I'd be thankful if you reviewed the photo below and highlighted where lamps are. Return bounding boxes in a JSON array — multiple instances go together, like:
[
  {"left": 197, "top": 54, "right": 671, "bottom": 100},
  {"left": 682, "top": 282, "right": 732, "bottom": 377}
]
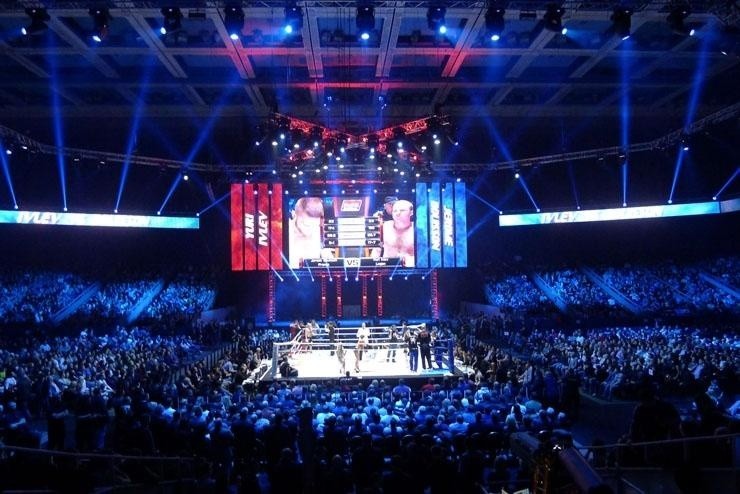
[{"left": 16, "top": 1, "right": 701, "bottom": 48}]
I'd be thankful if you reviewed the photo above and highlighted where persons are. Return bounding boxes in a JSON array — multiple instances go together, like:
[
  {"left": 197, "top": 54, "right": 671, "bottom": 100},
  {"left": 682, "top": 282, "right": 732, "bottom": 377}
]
[
  {"left": 371, "top": 195, "right": 400, "bottom": 223},
  {"left": 368, "top": 197, "right": 415, "bottom": 267},
  {"left": 357, "top": 334, "right": 365, "bottom": 361},
  {"left": 0, "top": 377, "right": 740, "bottom": 491},
  {"left": 334, "top": 341, "right": 348, "bottom": 372},
  {"left": 462, "top": 247, "right": 739, "bottom": 378},
  {"left": 356, "top": 322, "right": 371, "bottom": 354},
  {"left": 0, "top": 267, "right": 224, "bottom": 379},
  {"left": 324, "top": 320, "right": 336, "bottom": 357},
  {"left": 386, "top": 324, "right": 400, "bottom": 362},
  {"left": 287, "top": 194, "right": 338, "bottom": 268},
  {"left": 352, "top": 344, "right": 361, "bottom": 374},
  {"left": 223, "top": 316, "right": 322, "bottom": 381},
  {"left": 396, "top": 320, "right": 463, "bottom": 379}
]
